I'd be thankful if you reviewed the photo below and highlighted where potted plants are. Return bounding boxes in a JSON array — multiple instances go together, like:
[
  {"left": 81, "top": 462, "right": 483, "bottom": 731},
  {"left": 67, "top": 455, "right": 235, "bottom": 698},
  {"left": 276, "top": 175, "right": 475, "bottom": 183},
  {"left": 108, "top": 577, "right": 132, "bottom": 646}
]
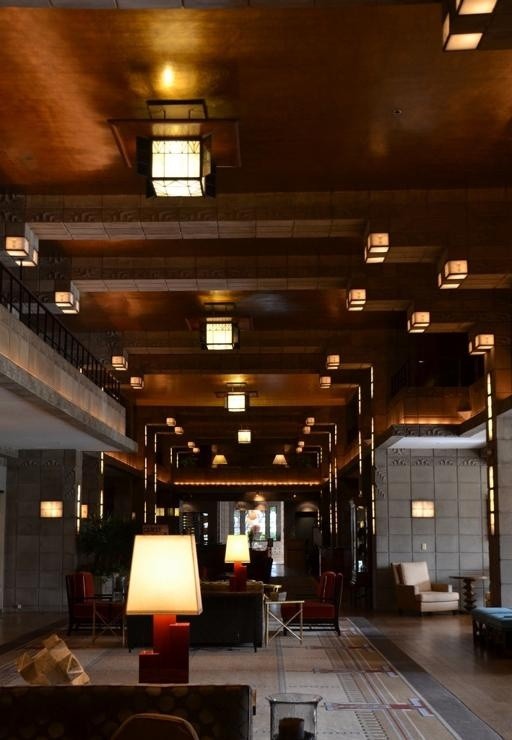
[{"left": 76, "top": 510, "right": 133, "bottom": 600}]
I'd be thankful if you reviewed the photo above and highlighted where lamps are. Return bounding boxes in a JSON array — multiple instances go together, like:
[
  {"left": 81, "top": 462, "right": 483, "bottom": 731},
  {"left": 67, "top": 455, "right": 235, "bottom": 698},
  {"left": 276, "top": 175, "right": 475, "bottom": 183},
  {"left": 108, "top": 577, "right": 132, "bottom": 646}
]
[
  {"left": 123, "top": 533, "right": 205, "bottom": 684},
  {"left": 4, "top": 221, "right": 44, "bottom": 273},
  {"left": 51, "top": 278, "right": 84, "bottom": 318},
  {"left": 223, "top": 532, "right": 252, "bottom": 580},
  {"left": 111, "top": 346, "right": 131, "bottom": 373},
  {"left": 441, "top": 0, "right": 500, "bottom": 53},
  {"left": 220, "top": 381, "right": 254, "bottom": 414},
  {"left": 132, "top": 101, "right": 221, "bottom": 201},
  {"left": 466, "top": 324, "right": 496, "bottom": 360},
  {"left": 296, "top": 354, "right": 345, "bottom": 457},
  {"left": 212, "top": 451, "right": 288, "bottom": 466},
  {"left": 197, "top": 300, "right": 241, "bottom": 353},
  {"left": 434, "top": 241, "right": 471, "bottom": 294},
  {"left": 405, "top": 301, "right": 432, "bottom": 339},
  {"left": 236, "top": 422, "right": 253, "bottom": 446},
  {"left": 343, "top": 279, "right": 366, "bottom": 313},
  {"left": 357, "top": 216, "right": 393, "bottom": 268},
  {"left": 130, "top": 369, "right": 201, "bottom": 456}
]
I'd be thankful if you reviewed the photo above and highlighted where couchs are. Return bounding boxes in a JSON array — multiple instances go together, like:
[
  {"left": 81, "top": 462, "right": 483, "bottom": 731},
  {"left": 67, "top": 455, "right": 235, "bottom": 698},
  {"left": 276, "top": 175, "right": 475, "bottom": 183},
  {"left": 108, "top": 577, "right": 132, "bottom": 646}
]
[{"left": 0, "top": 682, "right": 258, "bottom": 739}]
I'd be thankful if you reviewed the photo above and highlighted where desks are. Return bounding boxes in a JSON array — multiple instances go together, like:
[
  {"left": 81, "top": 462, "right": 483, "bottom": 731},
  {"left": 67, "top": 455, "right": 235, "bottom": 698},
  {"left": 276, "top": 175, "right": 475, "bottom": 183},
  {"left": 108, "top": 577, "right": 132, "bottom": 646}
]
[{"left": 448, "top": 571, "right": 489, "bottom": 613}]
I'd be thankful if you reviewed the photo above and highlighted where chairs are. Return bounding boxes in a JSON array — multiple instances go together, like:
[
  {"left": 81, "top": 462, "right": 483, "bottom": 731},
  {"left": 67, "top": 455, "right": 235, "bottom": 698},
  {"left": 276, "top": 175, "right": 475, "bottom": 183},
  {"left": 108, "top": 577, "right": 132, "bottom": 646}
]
[
  {"left": 63, "top": 567, "right": 345, "bottom": 654},
  {"left": 388, "top": 560, "right": 460, "bottom": 617},
  {"left": 470, "top": 603, "right": 511, "bottom": 657},
  {"left": 112, "top": 712, "right": 199, "bottom": 739}
]
